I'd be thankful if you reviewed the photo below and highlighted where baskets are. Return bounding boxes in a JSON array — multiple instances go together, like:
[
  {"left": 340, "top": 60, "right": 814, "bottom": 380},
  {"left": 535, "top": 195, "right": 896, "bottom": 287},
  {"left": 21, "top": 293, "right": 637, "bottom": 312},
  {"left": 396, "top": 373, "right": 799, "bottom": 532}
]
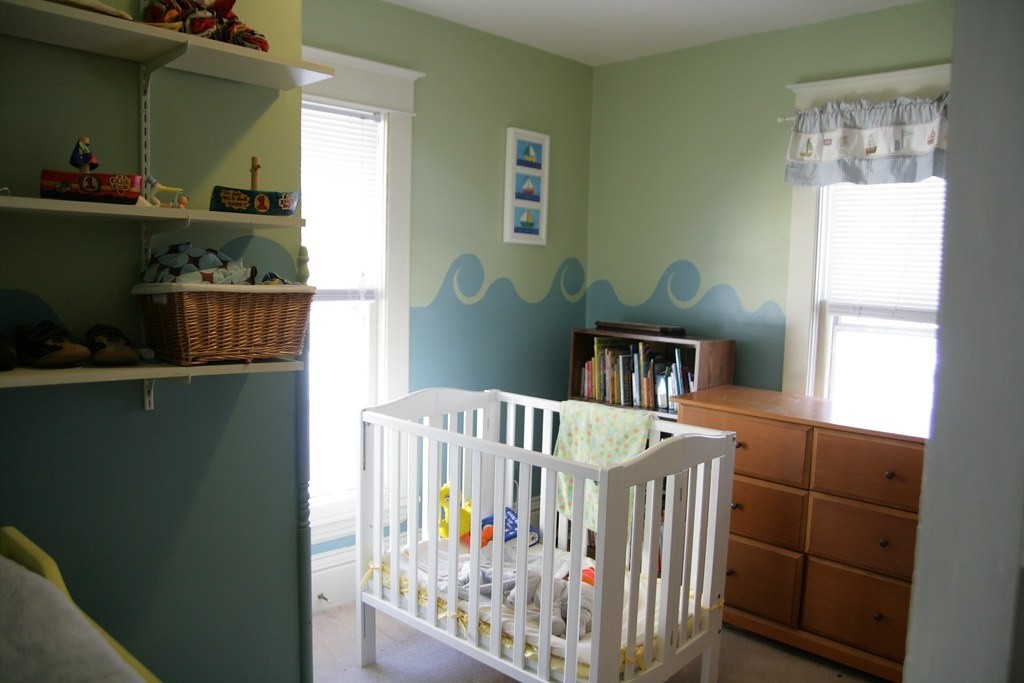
[{"left": 130, "top": 282, "right": 316, "bottom": 368}]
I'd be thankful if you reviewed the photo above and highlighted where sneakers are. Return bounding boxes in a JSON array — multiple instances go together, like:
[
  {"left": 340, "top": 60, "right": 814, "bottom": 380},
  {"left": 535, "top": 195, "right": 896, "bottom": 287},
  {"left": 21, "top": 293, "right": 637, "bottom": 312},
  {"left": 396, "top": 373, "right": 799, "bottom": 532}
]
[
  {"left": 16, "top": 321, "right": 92, "bottom": 368},
  {"left": 86, "top": 325, "right": 141, "bottom": 365}
]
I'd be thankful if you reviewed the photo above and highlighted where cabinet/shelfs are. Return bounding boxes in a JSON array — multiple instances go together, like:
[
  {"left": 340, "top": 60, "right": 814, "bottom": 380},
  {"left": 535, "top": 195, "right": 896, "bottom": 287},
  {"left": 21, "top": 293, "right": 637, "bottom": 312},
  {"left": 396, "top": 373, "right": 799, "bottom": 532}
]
[
  {"left": 1, "top": 1, "right": 335, "bottom": 411},
  {"left": 566, "top": 319, "right": 736, "bottom": 420},
  {"left": 671, "top": 381, "right": 930, "bottom": 683}
]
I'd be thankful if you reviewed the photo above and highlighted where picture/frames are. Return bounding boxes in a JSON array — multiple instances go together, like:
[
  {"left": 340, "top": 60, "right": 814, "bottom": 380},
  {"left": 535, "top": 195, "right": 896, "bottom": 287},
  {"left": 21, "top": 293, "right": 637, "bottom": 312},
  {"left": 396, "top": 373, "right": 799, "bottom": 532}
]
[{"left": 501, "top": 128, "right": 549, "bottom": 248}]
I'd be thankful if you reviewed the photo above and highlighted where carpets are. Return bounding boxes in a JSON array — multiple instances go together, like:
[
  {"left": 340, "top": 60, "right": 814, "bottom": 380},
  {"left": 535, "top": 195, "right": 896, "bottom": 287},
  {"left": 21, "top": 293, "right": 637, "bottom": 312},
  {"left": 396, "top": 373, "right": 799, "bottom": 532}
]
[{"left": 312, "top": 546, "right": 887, "bottom": 683}]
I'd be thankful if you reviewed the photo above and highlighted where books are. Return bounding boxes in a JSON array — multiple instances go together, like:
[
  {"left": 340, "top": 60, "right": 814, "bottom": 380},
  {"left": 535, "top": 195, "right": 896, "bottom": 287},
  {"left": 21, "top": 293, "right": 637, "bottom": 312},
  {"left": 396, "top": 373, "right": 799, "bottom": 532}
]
[{"left": 576, "top": 337, "right": 697, "bottom": 411}]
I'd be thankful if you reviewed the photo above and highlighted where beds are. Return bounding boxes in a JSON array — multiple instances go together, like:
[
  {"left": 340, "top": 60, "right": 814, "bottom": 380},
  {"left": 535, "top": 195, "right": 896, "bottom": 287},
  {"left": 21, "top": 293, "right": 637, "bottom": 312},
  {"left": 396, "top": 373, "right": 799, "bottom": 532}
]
[{"left": 357, "top": 386, "right": 738, "bottom": 683}]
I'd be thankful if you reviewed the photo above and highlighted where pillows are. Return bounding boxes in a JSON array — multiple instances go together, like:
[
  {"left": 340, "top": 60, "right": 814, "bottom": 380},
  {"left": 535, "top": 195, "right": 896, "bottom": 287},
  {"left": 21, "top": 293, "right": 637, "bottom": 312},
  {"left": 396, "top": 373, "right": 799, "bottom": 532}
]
[{"left": 481, "top": 506, "right": 540, "bottom": 553}]
